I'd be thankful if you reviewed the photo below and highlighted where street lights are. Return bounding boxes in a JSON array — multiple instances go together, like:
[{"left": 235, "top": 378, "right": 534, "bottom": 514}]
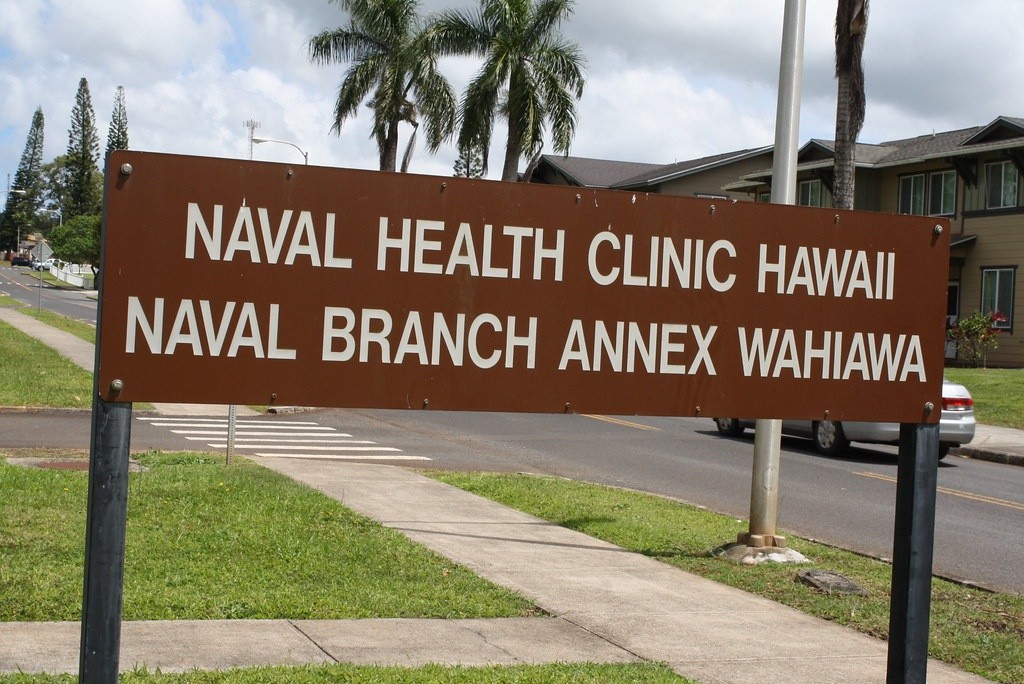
[{"left": 251, "top": 137, "right": 308, "bottom": 165}]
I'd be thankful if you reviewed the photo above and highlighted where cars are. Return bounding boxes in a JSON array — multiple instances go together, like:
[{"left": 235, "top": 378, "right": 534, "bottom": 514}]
[
  {"left": 33, "top": 257, "right": 57, "bottom": 272},
  {"left": 713, "top": 373, "right": 977, "bottom": 464},
  {"left": 11, "top": 257, "right": 30, "bottom": 267}
]
